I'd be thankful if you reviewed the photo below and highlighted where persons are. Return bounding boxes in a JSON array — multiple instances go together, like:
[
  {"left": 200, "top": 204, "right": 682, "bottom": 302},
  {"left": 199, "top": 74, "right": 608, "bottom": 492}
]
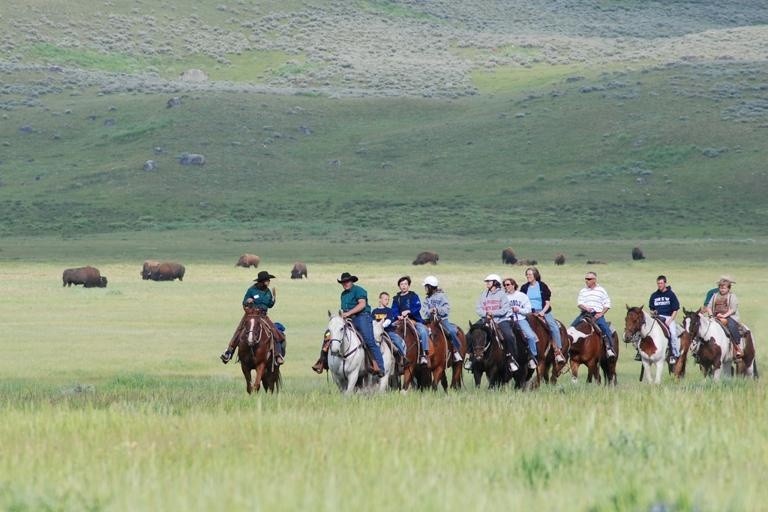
[
  {"left": 220, "top": 271, "right": 284, "bottom": 364},
  {"left": 702, "top": 276, "right": 736, "bottom": 313},
  {"left": 649, "top": 275, "right": 680, "bottom": 366},
  {"left": 312, "top": 266, "right": 567, "bottom": 377},
  {"left": 569, "top": 272, "right": 617, "bottom": 361},
  {"left": 706, "top": 280, "right": 744, "bottom": 358}
]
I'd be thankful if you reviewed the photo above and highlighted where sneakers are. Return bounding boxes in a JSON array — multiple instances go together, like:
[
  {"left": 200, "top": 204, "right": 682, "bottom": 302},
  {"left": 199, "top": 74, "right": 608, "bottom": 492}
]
[
  {"left": 275, "top": 353, "right": 285, "bottom": 367},
  {"left": 526, "top": 357, "right": 538, "bottom": 370},
  {"left": 605, "top": 349, "right": 616, "bottom": 361},
  {"left": 418, "top": 352, "right": 430, "bottom": 366},
  {"left": 452, "top": 350, "right": 465, "bottom": 364},
  {"left": 219, "top": 347, "right": 234, "bottom": 364},
  {"left": 552, "top": 352, "right": 566, "bottom": 365},
  {"left": 506, "top": 360, "right": 521, "bottom": 375}
]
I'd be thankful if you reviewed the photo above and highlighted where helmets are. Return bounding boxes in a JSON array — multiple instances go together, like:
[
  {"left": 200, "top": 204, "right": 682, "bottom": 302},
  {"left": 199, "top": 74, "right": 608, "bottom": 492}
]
[
  {"left": 421, "top": 275, "right": 440, "bottom": 287},
  {"left": 483, "top": 273, "right": 503, "bottom": 285}
]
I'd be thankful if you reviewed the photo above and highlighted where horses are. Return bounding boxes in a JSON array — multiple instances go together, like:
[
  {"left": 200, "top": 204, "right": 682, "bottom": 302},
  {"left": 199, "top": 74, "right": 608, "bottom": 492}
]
[
  {"left": 680, "top": 307, "right": 759, "bottom": 383},
  {"left": 567, "top": 316, "right": 620, "bottom": 386},
  {"left": 237, "top": 302, "right": 284, "bottom": 393},
  {"left": 322, "top": 307, "right": 468, "bottom": 396},
  {"left": 464, "top": 314, "right": 573, "bottom": 386},
  {"left": 621, "top": 302, "right": 684, "bottom": 385}
]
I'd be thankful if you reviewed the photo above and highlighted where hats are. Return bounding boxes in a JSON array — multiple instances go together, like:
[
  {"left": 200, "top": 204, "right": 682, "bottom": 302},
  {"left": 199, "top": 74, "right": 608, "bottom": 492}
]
[
  {"left": 336, "top": 272, "right": 358, "bottom": 283},
  {"left": 252, "top": 270, "right": 276, "bottom": 282}
]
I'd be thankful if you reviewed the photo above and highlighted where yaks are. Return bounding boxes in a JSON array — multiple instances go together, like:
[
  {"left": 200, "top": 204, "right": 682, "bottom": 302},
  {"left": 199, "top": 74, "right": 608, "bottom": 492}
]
[
  {"left": 412, "top": 251, "right": 440, "bottom": 266},
  {"left": 61, "top": 266, "right": 108, "bottom": 288},
  {"left": 141, "top": 259, "right": 186, "bottom": 281},
  {"left": 234, "top": 253, "right": 261, "bottom": 269},
  {"left": 502, "top": 247, "right": 538, "bottom": 265},
  {"left": 554, "top": 254, "right": 566, "bottom": 265},
  {"left": 632, "top": 248, "right": 645, "bottom": 261},
  {"left": 290, "top": 263, "right": 308, "bottom": 278}
]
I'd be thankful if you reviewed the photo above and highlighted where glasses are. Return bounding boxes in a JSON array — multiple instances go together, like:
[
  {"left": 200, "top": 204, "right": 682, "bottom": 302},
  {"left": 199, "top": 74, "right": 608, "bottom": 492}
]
[
  {"left": 504, "top": 284, "right": 511, "bottom": 287},
  {"left": 584, "top": 277, "right": 594, "bottom": 281}
]
